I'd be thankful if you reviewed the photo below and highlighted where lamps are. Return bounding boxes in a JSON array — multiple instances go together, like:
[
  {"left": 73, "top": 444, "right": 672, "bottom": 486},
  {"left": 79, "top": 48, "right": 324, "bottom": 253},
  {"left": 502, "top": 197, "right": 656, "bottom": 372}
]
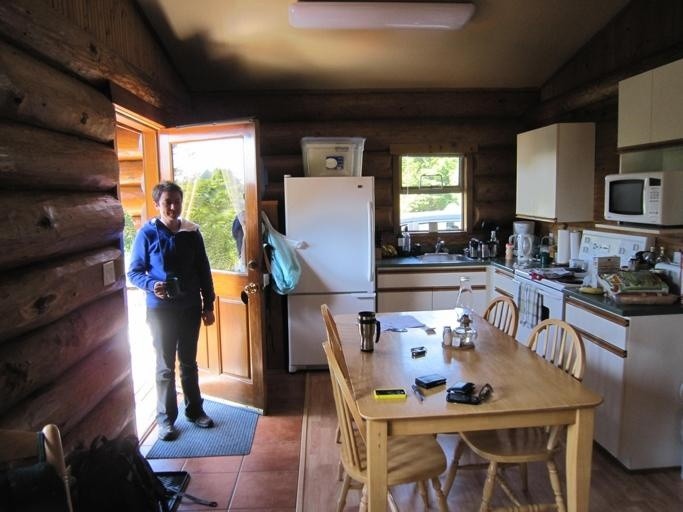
[{"left": 285, "top": 0, "right": 477, "bottom": 34}]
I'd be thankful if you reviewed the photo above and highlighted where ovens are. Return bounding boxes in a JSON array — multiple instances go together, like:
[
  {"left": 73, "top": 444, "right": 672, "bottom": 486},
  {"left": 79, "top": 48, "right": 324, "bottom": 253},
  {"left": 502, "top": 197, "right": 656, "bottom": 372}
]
[{"left": 512, "top": 275, "right": 564, "bottom": 368}]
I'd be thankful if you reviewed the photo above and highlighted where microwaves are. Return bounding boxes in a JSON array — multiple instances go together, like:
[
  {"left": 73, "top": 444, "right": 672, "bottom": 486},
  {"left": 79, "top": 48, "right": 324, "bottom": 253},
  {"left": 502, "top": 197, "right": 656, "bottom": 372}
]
[{"left": 602, "top": 170, "right": 682, "bottom": 227}]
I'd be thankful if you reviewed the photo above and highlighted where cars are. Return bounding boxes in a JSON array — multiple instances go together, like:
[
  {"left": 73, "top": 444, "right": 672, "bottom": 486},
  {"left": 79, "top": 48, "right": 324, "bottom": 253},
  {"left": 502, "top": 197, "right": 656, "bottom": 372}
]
[{"left": 399, "top": 214, "right": 460, "bottom": 232}]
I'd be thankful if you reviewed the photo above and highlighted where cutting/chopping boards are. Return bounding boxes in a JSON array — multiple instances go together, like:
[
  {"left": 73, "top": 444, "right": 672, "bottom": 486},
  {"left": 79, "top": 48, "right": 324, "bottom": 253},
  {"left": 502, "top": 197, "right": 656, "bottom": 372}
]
[{"left": 611, "top": 293, "right": 678, "bottom": 305}]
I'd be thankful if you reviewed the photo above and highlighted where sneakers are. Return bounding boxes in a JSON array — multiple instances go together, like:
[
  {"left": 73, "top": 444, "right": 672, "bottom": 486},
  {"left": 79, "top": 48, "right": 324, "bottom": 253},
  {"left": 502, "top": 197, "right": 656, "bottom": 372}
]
[
  {"left": 185, "top": 406, "right": 213, "bottom": 427},
  {"left": 159, "top": 422, "right": 176, "bottom": 439}
]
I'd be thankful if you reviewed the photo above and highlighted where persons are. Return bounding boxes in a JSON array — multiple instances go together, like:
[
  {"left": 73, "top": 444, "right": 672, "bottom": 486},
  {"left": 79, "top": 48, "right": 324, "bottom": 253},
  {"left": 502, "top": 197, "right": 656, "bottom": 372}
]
[
  {"left": 231, "top": 193, "right": 249, "bottom": 272},
  {"left": 126, "top": 180, "right": 216, "bottom": 443}
]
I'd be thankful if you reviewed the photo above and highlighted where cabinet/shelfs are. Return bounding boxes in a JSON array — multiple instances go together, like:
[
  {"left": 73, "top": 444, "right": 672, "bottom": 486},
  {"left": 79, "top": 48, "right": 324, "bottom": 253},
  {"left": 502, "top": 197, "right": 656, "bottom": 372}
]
[
  {"left": 561, "top": 297, "right": 681, "bottom": 478},
  {"left": 616, "top": 58, "right": 682, "bottom": 153},
  {"left": 514, "top": 122, "right": 596, "bottom": 225},
  {"left": 375, "top": 262, "right": 489, "bottom": 314},
  {"left": 490, "top": 263, "right": 521, "bottom": 338}
]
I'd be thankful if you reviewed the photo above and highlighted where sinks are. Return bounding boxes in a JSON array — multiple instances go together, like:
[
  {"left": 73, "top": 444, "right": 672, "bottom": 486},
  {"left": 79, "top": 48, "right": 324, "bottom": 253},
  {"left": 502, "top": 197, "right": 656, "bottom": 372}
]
[{"left": 415, "top": 253, "right": 477, "bottom": 264}]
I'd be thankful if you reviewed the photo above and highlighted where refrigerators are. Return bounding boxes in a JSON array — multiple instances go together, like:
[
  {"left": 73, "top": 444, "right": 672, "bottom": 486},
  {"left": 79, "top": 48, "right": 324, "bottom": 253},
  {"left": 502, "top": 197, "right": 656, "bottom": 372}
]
[{"left": 282, "top": 174, "right": 376, "bottom": 373}]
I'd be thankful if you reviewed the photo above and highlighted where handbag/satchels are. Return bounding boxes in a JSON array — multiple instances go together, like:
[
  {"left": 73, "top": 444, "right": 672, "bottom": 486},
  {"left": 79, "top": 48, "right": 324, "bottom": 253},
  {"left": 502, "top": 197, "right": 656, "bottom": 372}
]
[{"left": 7, "top": 462, "right": 69, "bottom": 512}]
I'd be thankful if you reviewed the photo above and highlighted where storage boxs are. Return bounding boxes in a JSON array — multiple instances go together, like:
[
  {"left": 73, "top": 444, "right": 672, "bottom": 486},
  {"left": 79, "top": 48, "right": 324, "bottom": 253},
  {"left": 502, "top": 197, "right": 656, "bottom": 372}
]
[{"left": 298, "top": 134, "right": 366, "bottom": 177}]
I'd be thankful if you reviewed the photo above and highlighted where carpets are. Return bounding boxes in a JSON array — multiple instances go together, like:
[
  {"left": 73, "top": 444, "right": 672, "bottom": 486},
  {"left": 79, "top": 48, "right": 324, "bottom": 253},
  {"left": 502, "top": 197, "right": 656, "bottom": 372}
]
[{"left": 144, "top": 386, "right": 265, "bottom": 460}]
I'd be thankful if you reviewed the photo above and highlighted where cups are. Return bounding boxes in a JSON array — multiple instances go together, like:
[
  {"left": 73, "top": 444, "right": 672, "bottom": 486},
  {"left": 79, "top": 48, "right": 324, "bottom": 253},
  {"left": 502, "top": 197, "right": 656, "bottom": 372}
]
[
  {"left": 162, "top": 277, "right": 180, "bottom": 300},
  {"left": 357, "top": 310, "right": 381, "bottom": 352},
  {"left": 540, "top": 252, "right": 550, "bottom": 264},
  {"left": 568, "top": 259, "right": 576, "bottom": 268}
]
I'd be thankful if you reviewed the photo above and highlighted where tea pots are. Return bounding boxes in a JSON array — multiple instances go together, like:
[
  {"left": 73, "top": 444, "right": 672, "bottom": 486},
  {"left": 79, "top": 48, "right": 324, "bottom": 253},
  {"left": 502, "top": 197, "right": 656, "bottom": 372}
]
[{"left": 538, "top": 235, "right": 557, "bottom": 261}]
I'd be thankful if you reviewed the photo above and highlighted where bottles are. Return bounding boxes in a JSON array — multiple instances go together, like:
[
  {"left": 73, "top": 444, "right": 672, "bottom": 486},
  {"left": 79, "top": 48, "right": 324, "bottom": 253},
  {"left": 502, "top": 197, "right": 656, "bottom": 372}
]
[
  {"left": 396, "top": 225, "right": 411, "bottom": 255},
  {"left": 468, "top": 241, "right": 488, "bottom": 259},
  {"left": 435, "top": 241, "right": 440, "bottom": 254},
  {"left": 646, "top": 246, "right": 669, "bottom": 266},
  {"left": 489, "top": 231, "right": 497, "bottom": 258},
  {"left": 504, "top": 243, "right": 513, "bottom": 260},
  {"left": 455, "top": 278, "right": 477, "bottom": 328},
  {"left": 442, "top": 326, "right": 453, "bottom": 346}
]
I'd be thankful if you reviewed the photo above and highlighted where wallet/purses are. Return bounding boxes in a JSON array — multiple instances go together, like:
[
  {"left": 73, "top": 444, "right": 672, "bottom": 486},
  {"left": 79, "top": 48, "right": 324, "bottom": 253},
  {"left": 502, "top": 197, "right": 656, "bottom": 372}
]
[{"left": 447, "top": 383, "right": 476, "bottom": 393}]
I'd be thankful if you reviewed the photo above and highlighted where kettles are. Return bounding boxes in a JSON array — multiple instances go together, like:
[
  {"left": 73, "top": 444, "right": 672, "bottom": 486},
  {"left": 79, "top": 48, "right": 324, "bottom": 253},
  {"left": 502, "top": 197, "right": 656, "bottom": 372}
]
[{"left": 517, "top": 233, "right": 536, "bottom": 261}]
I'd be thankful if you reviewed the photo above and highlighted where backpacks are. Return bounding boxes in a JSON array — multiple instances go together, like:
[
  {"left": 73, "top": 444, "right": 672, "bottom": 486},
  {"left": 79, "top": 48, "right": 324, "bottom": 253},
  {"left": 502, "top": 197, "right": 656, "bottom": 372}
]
[{"left": 90, "top": 436, "right": 167, "bottom": 500}]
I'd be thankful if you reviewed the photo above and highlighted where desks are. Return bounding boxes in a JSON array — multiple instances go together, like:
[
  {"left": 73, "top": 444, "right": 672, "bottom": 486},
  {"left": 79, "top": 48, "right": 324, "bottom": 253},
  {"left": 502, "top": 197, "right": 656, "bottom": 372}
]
[{"left": 333, "top": 306, "right": 603, "bottom": 512}]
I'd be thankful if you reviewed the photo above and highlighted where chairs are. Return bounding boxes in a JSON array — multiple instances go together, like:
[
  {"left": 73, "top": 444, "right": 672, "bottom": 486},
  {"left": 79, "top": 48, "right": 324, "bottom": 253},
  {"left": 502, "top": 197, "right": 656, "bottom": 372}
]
[
  {"left": 319, "top": 338, "right": 451, "bottom": 510},
  {"left": 1, "top": 424, "right": 78, "bottom": 512},
  {"left": 318, "top": 302, "right": 438, "bottom": 449},
  {"left": 439, "top": 316, "right": 588, "bottom": 510},
  {"left": 480, "top": 295, "right": 521, "bottom": 342}
]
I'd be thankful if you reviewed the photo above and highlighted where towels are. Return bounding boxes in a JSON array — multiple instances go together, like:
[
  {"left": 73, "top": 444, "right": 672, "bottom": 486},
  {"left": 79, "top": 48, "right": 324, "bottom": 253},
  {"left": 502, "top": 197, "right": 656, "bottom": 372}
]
[{"left": 514, "top": 282, "right": 542, "bottom": 330}]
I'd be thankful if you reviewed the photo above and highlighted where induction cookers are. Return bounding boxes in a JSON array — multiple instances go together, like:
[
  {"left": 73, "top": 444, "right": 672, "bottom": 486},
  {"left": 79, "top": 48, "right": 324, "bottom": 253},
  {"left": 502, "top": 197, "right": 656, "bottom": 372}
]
[{"left": 515, "top": 267, "right": 589, "bottom": 286}]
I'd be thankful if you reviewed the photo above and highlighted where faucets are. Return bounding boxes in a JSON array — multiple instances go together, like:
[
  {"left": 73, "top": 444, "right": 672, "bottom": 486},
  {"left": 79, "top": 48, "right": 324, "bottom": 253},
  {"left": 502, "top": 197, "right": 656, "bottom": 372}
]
[{"left": 434, "top": 239, "right": 446, "bottom": 254}]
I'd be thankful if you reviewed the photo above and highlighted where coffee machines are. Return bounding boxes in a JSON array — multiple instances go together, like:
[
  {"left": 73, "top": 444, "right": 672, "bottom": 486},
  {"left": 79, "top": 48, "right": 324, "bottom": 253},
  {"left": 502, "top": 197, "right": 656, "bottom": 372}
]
[{"left": 508, "top": 220, "right": 535, "bottom": 256}]
